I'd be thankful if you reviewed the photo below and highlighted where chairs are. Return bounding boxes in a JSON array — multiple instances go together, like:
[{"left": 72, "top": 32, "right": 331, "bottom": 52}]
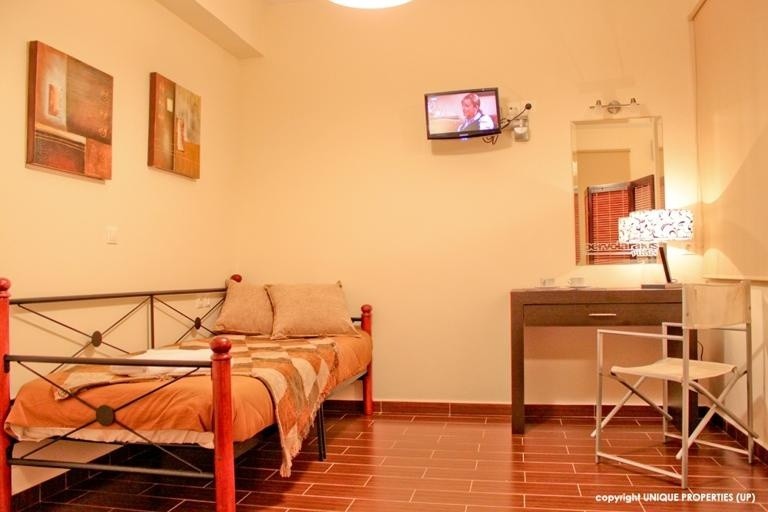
[{"left": 591, "top": 281, "right": 759, "bottom": 489}]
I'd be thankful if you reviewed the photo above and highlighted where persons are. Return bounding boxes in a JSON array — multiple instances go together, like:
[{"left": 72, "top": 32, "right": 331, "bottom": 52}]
[{"left": 457, "top": 92, "right": 494, "bottom": 133}]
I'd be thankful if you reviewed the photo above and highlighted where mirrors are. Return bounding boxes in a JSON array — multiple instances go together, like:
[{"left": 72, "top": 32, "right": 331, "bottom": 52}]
[{"left": 571, "top": 115, "right": 667, "bottom": 266}]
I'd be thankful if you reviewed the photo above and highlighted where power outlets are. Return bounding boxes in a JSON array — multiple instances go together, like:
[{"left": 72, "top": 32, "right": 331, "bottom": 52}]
[{"left": 507, "top": 103, "right": 532, "bottom": 143}]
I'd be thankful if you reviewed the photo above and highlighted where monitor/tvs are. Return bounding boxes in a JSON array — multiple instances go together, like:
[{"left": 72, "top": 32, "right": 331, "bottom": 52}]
[{"left": 424, "top": 88, "right": 501, "bottom": 140}]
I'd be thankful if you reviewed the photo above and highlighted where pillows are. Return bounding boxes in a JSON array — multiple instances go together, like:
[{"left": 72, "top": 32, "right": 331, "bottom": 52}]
[
  {"left": 264, "top": 280, "right": 361, "bottom": 340},
  {"left": 211, "top": 279, "right": 272, "bottom": 335}
]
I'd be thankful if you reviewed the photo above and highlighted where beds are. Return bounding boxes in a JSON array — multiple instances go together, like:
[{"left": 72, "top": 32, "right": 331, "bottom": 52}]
[{"left": 0, "top": 274, "right": 372, "bottom": 512}]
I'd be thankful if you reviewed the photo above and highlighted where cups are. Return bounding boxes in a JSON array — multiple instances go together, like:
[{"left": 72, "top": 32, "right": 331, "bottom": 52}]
[
  {"left": 566, "top": 277, "right": 585, "bottom": 287},
  {"left": 540, "top": 277, "right": 556, "bottom": 287}
]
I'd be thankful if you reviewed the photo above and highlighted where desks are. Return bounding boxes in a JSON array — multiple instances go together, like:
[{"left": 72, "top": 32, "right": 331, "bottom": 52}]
[{"left": 511, "top": 289, "right": 697, "bottom": 436}]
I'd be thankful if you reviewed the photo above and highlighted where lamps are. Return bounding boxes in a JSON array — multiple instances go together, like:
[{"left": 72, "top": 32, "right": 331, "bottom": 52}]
[
  {"left": 590, "top": 98, "right": 642, "bottom": 119},
  {"left": 618, "top": 217, "right": 629, "bottom": 245},
  {"left": 630, "top": 209, "right": 693, "bottom": 286}
]
